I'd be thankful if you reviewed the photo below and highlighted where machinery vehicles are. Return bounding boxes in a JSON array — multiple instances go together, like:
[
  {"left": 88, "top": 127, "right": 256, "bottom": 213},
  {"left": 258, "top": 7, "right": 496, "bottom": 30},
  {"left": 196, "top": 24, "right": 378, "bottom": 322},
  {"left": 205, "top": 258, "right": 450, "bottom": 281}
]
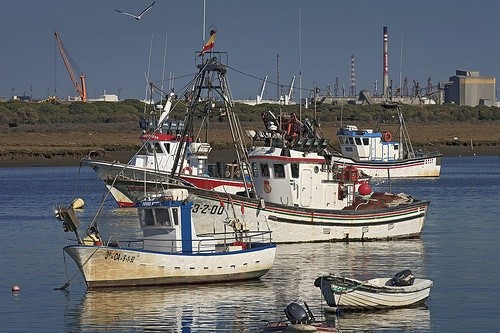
[{"left": 53, "top": 30, "right": 88, "bottom": 104}]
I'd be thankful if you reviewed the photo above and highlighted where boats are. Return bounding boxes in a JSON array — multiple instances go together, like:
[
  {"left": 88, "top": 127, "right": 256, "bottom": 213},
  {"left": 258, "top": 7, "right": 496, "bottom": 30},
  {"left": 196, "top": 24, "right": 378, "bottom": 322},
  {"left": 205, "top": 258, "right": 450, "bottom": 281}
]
[{"left": 313, "top": 268, "right": 435, "bottom": 312}]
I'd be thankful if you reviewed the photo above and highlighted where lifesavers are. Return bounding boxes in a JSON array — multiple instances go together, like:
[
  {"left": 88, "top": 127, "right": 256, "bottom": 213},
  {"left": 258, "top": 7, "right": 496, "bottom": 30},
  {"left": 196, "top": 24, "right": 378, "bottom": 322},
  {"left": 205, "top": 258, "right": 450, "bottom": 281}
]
[
  {"left": 181, "top": 166, "right": 192, "bottom": 176},
  {"left": 382, "top": 131, "right": 392, "bottom": 142}
]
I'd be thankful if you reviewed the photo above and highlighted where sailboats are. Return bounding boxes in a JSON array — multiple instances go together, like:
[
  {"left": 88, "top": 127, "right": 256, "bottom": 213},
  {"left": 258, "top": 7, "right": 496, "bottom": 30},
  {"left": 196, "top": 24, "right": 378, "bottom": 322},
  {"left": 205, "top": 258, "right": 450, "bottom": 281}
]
[
  {"left": 162, "top": 25, "right": 431, "bottom": 240},
  {"left": 52, "top": 150, "right": 278, "bottom": 291},
  {"left": 88, "top": 73, "right": 257, "bottom": 208},
  {"left": 329, "top": 91, "right": 444, "bottom": 179}
]
